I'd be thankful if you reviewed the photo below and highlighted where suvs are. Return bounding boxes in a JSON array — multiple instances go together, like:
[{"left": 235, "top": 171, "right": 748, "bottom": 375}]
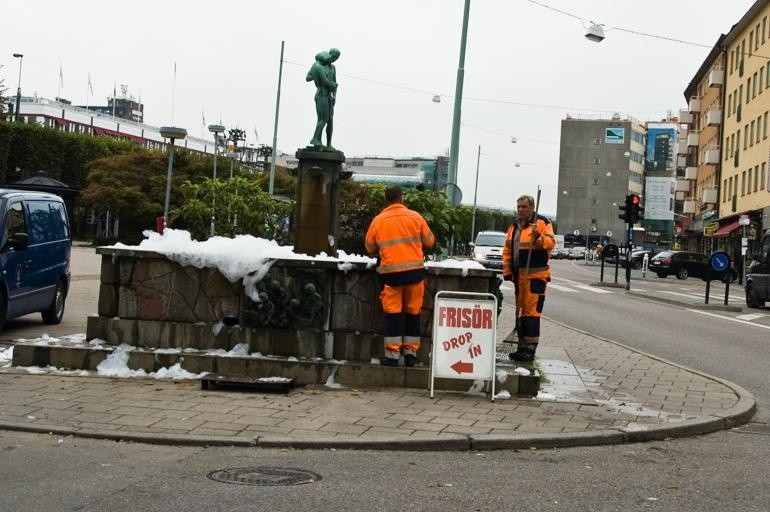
[{"left": 468, "top": 231, "right": 507, "bottom": 273}]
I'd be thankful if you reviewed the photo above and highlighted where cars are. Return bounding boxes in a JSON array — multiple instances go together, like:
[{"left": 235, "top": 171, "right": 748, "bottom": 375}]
[
  {"left": 550, "top": 247, "right": 661, "bottom": 270},
  {"left": 744, "top": 227, "right": 770, "bottom": 308},
  {"left": 649, "top": 250, "right": 737, "bottom": 284}
]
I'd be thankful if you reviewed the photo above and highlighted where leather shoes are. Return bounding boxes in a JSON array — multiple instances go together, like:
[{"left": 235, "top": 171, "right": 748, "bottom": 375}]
[
  {"left": 404, "top": 355, "right": 417, "bottom": 366},
  {"left": 509, "top": 348, "right": 534, "bottom": 361},
  {"left": 380, "top": 358, "right": 398, "bottom": 366}
]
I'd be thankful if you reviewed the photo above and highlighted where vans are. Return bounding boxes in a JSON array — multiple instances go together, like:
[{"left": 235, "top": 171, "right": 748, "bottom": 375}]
[{"left": 0, "top": 189, "right": 72, "bottom": 333}]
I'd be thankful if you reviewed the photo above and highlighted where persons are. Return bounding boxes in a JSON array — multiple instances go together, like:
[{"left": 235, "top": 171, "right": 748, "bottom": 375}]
[
  {"left": 502, "top": 195, "right": 555, "bottom": 360},
  {"left": 306, "top": 48, "right": 340, "bottom": 148},
  {"left": 243, "top": 274, "right": 324, "bottom": 329},
  {"left": 364, "top": 185, "right": 435, "bottom": 366}
]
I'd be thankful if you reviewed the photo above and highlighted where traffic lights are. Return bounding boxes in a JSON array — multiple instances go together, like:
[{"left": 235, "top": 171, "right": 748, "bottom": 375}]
[
  {"left": 634, "top": 200, "right": 645, "bottom": 223},
  {"left": 618, "top": 196, "right": 631, "bottom": 223},
  {"left": 627, "top": 193, "right": 640, "bottom": 225}
]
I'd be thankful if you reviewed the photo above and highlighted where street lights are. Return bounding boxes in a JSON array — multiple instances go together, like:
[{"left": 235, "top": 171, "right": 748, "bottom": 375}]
[
  {"left": 13, "top": 53, "right": 23, "bottom": 122},
  {"left": 207, "top": 124, "right": 225, "bottom": 237},
  {"left": 227, "top": 153, "right": 239, "bottom": 225},
  {"left": 159, "top": 127, "right": 187, "bottom": 229}
]
